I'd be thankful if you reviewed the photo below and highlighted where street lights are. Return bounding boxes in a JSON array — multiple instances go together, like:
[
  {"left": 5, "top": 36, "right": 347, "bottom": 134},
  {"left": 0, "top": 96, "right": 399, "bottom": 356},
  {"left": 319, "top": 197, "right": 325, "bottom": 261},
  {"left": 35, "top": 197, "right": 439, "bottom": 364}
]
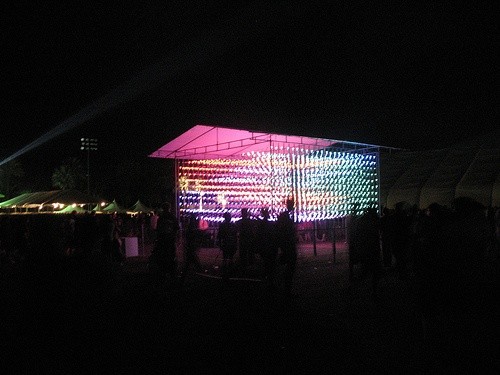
[{"left": 79, "top": 137, "right": 99, "bottom": 214}]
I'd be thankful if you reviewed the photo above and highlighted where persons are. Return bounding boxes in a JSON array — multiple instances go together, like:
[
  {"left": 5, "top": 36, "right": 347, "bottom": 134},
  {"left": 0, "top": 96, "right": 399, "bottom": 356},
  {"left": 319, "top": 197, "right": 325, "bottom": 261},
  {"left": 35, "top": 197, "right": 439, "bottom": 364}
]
[
  {"left": 155, "top": 202, "right": 180, "bottom": 269},
  {"left": 1, "top": 200, "right": 159, "bottom": 264},
  {"left": 345, "top": 199, "right": 500, "bottom": 270},
  {"left": 253, "top": 207, "right": 276, "bottom": 259},
  {"left": 217, "top": 211, "right": 238, "bottom": 270},
  {"left": 236, "top": 208, "right": 256, "bottom": 266},
  {"left": 276, "top": 196, "right": 299, "bottom": 293},
  {"left": 181, "top": 216, "right": 209, "bottom": 272}
]
